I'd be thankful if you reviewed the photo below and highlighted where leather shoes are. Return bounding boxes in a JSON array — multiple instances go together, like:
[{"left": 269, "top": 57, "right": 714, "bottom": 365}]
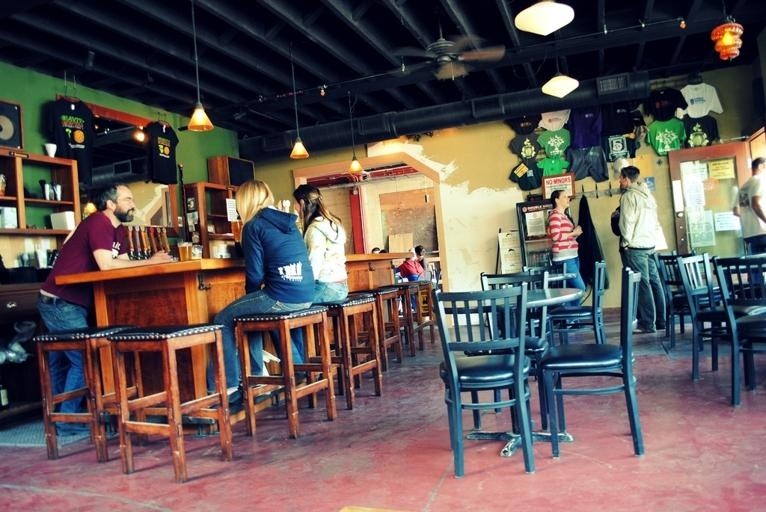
[{"left": 56, "top": 422, "right": 90, "bottom": 435}]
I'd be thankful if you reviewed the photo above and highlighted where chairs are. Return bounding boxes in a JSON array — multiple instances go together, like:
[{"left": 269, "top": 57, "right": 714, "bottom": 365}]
[
  {"left": 479, "top": 262, "right": 611, "bottom": 384},
  {"left": 545, "top": 267, "right": 644, "bottom": 460},
  {"left": 431, "top": 281, "right": 539, "bottom": 477},
  {"left": 655, "top": 248, "right": 766, "bottom": 406}
]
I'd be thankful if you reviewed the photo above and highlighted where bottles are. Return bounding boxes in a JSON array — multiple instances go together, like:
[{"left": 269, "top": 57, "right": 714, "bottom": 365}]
[{"left": 528, "top": 247, "right": 550, "bottom": 267}]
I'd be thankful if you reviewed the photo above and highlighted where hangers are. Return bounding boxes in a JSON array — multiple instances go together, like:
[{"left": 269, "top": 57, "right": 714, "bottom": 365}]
[
  {"left": 64, "top": 82, "right": 80, "bottom": 101},
  {"left": 156, "top": 110, "right": 169, "bottom": 125}
]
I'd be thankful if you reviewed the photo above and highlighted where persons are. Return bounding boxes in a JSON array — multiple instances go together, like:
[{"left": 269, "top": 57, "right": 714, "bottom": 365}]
[
  {"left": 371, "top": 245, "right": 428, "bottom": 310},
  {"left": 733, "top": 157, "right": 766, "bottom": 254},
  {"left": 610, "top": 185, "right": 628, "bottom": 270},
  {"left": 208, "top": 179, "right": 313, "bottom": 402},
  {"left": 611, "top": 166, "right": 665, "bottom": 332},
  {"left": 293, "top": 183, "right": 348, "bottom": 305},
  {"left": 35, "top": 181, "right": 172, "bottom": 435},
  {"left": 548, "top": 190, "right": 585, "bottom": 328}
]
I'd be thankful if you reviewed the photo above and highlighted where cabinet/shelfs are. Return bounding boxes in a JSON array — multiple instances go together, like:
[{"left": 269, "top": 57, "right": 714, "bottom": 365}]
[
  {"left": 181, "top": 155, "right": 256, "bottom": 258},
  {"left": 0, "top": 279, "right": 47, "bottom": 429},
  {"left": 0, "top": 146, "right": 81, "bottom": 237}
]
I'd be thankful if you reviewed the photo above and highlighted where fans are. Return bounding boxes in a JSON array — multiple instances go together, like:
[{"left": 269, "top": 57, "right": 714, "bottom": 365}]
[{"left": 387, "top": 27, "right": 504, "bottom": 79}]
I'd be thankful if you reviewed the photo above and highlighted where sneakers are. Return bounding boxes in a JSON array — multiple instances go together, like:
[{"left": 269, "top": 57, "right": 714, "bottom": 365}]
[{"left": 632, "top": 327, "right": 652, "bottom": 334}]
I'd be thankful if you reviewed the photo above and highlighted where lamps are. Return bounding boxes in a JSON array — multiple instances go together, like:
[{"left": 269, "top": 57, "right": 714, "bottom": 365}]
[
  {"left": 514, "top": 0, "right": 575, "bottom": 36},
  {"left": 289, "top": 55, "right": 311, "bottom": 160},
  {"left": 187, "top": 2, "right": 215, "bottom": 136},
  {"left": 541, "top": 69, "right": 581, "bottom": 100},
  {"left": 347, "top": 92, "right": 363, "bottom": 175},
  {"left": 708, "top": 10, "right": 744, "bottom": 63}
]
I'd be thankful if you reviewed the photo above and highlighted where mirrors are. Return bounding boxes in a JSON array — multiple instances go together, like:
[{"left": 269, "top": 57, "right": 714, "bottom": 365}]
[{"left": 51, "top": 93, "right": 178, "bottom": 237}]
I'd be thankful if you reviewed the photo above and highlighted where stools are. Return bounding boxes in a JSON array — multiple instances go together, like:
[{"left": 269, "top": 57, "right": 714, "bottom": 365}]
[
  {"left": 343, "top": 285, "right": 403, "bottom": 371},
  {"left": 310, "top": 288, "right": 383, "bottom": 412},
  {"left": 387, "top": 279, "right": 436, "bottom": 351},
  {"left": 111, "top": 324, "right": 232, "bottom": 485},
  {"left": 376, "top": 282, "right": 424, "bottom": 360},
  {"left": 230, "top": 304, "right": 339, "bottom": 441},
  {"left": 30, "top": 324, "right": 146, "bottom": 463}
]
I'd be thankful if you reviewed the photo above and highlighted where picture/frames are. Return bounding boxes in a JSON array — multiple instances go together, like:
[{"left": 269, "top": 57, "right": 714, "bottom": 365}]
[{"left": 0, "top": 98, "right": 28, "bottom": 152}]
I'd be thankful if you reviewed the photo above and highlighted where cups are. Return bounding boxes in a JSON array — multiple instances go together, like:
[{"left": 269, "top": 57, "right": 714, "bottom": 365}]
[
  {"left": 192, "top": 245, "right": 202, "bottom": 259},
  {"left": 20, "top": 253, "right": 29, "bottom": 267},
  {"left": 177, "top": 241, "right": 192, "bottom": 262},
  {"left": 44, "top": 143, "right": 57, "bottom": 159},
  {"left": 34, "top": 249, "right": 47, "bottom": 268}
]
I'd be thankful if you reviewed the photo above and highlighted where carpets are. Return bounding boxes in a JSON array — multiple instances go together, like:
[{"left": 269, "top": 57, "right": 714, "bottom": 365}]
[{"left": 0, "top": 408, "right": 102, "bottom": 449}]
